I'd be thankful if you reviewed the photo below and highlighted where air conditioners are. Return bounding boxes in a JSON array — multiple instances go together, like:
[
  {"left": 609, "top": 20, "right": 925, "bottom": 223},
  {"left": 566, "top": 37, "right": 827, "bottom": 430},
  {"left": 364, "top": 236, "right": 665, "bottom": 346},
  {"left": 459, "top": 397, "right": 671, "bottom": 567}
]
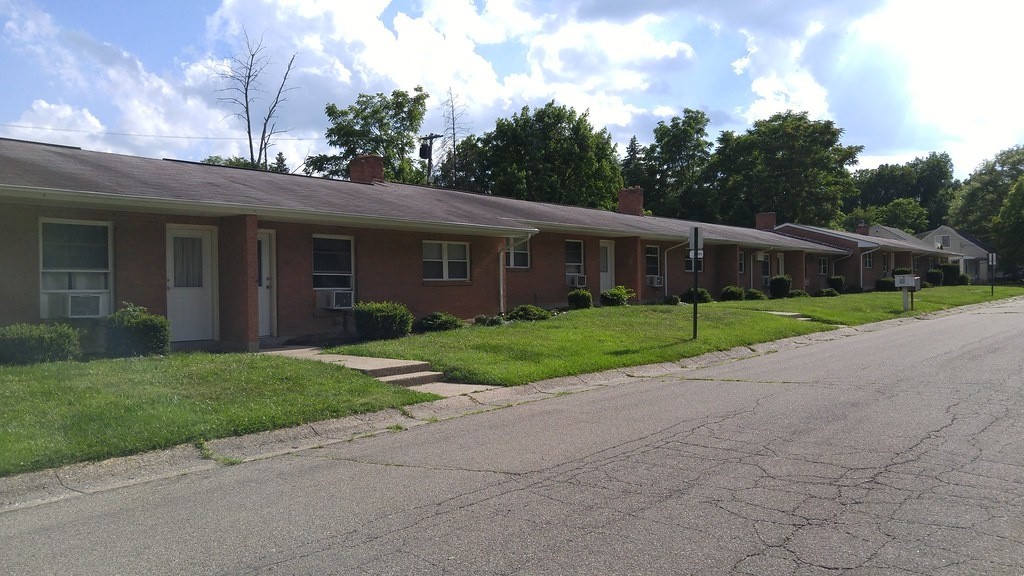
[
  {"left": 321, "top": 290, "right": 354, "bottom": 311},
  {"left": 56, "top": 292, "right": 100, "bottom": 318},
  {"left": 567, "top": 273, "right": 586, "bottom": 287},
  {"left": 646, "top": 274, "right": 664, "bottom": 288}
]
